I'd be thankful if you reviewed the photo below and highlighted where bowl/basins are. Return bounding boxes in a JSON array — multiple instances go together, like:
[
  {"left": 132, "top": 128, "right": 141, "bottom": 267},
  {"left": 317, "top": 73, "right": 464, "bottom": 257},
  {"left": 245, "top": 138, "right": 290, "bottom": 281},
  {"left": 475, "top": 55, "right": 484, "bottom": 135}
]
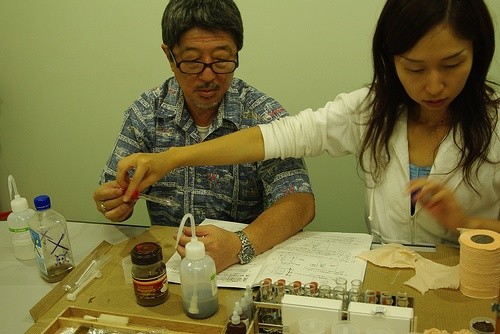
[{"left": 470, "top": 316, "right": 495, "bottom": 334}]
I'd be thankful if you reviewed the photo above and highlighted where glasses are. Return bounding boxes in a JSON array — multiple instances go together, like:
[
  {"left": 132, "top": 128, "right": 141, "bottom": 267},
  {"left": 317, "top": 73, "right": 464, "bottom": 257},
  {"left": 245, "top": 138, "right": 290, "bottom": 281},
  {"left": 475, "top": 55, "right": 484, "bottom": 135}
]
[{"left": 168, "top": 49, "right": 239, "bottom": 74}]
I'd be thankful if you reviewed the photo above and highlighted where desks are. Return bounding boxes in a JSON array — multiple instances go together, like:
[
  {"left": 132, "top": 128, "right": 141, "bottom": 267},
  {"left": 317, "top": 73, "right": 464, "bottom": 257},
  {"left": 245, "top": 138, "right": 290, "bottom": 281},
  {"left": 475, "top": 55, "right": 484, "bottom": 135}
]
[{"left": 0, "top": 222, "right": 500, "bottom": 334}]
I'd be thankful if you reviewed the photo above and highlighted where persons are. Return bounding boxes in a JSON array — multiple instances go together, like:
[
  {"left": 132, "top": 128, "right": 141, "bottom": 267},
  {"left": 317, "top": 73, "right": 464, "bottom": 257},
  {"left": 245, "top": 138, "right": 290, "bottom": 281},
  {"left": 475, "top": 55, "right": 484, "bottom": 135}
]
[
  {"left": 93, "top": 0, "right": 316, "bottom": 274},
  {"left": 116, "top": 0, "right": 500, "bottom": 248}
]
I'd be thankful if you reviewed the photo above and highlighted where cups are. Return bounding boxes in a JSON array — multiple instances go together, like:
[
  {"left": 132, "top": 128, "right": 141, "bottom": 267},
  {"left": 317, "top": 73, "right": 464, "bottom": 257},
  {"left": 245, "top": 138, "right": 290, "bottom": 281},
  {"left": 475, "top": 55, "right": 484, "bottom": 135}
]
[{"left": 298, "top": 318, "right": 396, "bottom": 334}]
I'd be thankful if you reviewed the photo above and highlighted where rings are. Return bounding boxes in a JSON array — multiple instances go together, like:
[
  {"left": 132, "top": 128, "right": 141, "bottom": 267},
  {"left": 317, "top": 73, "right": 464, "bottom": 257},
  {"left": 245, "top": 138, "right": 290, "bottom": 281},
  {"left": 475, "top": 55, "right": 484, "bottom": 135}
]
[{"left": 101, "top": 201, "right": 107, "bottom": 212}]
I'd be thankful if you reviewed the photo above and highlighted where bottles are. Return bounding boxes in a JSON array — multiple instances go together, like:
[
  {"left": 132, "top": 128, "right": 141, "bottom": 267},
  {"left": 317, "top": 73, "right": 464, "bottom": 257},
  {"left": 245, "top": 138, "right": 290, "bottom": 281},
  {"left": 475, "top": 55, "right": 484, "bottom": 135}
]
[
  {"left": 179, "top": 236, "right": 219, "bottom": 319},
  {"left": 27, "top": 195, "right": 76, "bottom": 284},
  {"left": 131, "top": 242, "right": 169, "bottom": 306},
  {"left": 7, "top": 194, "right": 37, "bottom": 261}
]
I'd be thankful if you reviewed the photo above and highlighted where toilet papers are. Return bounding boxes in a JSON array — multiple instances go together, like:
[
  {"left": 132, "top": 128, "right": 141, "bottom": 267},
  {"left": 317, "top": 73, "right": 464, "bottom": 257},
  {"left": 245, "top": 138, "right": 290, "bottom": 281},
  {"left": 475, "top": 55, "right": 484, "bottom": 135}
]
[{"left": 456, "top": 228, "right": 500, "bottom": 300}]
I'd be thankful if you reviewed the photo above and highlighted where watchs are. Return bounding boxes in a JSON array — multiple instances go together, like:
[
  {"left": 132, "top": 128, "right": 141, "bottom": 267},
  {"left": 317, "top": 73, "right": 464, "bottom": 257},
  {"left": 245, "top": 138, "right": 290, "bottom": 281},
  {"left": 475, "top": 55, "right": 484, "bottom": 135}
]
[{"left": 235, "top": 231, "right": 254, "bottom": 265}]
